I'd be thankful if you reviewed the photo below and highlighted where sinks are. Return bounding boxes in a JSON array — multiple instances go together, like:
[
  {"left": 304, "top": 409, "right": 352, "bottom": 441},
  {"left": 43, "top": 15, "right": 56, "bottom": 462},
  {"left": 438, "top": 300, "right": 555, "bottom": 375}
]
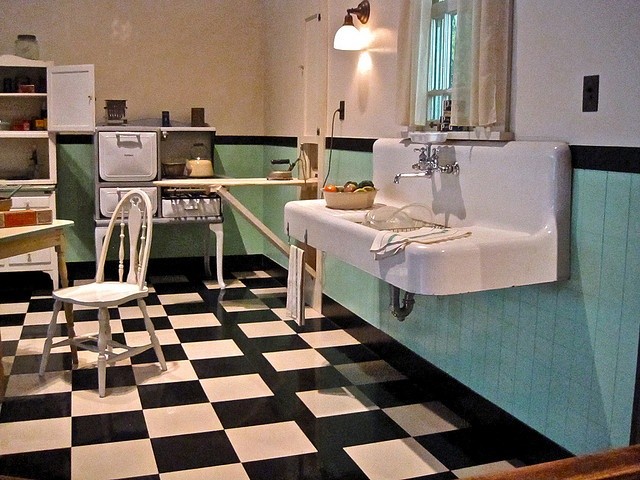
[{"left": 285, "top": 138, "right": 570, "bottom": 294}]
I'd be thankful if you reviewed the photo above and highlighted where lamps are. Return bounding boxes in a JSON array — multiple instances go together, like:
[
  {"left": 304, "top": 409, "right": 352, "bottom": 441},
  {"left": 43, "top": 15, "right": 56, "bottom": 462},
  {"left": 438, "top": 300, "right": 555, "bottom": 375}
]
[{"left": 334, "top": 0, "right": 371, "bottom": 52}]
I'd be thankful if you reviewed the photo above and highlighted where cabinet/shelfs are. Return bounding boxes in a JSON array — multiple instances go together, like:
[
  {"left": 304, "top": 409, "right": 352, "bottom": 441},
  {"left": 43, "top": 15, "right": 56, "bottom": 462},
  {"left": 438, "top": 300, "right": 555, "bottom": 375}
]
[
  {"left": 154, "top": 134, "right": 323, "bottom": 314},
  {"left": 0, "top": 54, "right": 96, "bottom": 289},
  {"left": 91, "top": 115, "right": 228, "bottom": 289}
]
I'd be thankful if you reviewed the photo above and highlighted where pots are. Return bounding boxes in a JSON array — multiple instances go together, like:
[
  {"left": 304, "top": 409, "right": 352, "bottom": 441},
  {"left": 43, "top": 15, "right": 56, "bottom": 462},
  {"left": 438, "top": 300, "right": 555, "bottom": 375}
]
[{"left": 1, "top": 196, "right": 13, "bottom": 211}]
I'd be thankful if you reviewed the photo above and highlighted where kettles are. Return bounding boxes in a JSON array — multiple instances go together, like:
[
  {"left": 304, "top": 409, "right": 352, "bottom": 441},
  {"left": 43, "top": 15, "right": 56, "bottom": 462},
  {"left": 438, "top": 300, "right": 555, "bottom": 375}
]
[{"left": 183, "top": 142, "right": 215, "bottom": 177}]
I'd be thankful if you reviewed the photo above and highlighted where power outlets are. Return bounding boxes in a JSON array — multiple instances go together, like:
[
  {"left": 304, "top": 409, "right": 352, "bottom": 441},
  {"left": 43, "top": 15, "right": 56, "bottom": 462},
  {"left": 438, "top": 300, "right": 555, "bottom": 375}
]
[{"left": 339, "top": 101, "right": 344, "bottom": 120}]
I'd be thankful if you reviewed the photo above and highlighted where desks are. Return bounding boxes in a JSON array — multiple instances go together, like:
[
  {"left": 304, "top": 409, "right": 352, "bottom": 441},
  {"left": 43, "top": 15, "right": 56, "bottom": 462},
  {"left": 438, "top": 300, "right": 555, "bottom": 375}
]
[{"left": 1, "top": 219, "right": 80, "bottom": 378}]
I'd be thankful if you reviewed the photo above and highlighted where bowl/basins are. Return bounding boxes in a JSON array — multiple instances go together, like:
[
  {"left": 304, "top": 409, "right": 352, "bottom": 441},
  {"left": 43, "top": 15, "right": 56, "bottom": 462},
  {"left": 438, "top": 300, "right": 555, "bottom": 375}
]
[
  {"left": 319, "top": 185, "right": 379, "bottom": 209},
  {"left": 162, "top": 161, "right": 186, "bottom": 178}
]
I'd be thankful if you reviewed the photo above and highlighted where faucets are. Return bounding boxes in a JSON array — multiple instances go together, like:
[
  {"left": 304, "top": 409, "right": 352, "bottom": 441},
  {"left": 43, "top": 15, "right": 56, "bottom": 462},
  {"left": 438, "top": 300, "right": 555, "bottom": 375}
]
[{"left": 393, "top": 148, "right": 439, "bottom": 184}]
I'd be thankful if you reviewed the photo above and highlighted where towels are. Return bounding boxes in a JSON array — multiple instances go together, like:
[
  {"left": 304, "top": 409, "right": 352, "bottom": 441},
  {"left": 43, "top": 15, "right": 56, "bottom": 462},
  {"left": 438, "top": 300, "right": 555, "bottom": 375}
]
[
  {"left": 285, "top": 244, "right": 305, "bottom": 326},
  {"left": 369, "top": 226, "right": 472, "bottom": 261}
]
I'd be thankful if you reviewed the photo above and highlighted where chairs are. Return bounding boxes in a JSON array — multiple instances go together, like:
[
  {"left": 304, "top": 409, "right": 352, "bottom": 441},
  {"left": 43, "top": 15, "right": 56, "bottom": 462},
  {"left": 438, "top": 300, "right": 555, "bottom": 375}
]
[{"left": 39, "top": 189, "right": 168, "bottom": 399}]
